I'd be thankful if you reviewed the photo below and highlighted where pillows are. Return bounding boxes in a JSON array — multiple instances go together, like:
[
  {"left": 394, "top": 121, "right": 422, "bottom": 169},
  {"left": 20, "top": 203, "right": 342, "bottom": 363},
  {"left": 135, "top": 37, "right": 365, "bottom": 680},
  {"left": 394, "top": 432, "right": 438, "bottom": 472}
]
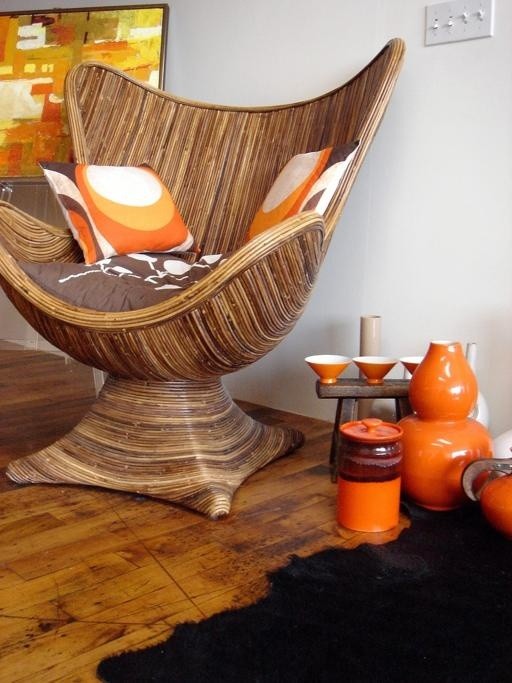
[
  {"left": 14, "top": 249, "right": 230, "bottom": 314},
  {"left": 36, "top": 158, "right": 203, "bottom": 266},
  {"left": 241, "top": 140, "right": 362, "bottom": 254}
]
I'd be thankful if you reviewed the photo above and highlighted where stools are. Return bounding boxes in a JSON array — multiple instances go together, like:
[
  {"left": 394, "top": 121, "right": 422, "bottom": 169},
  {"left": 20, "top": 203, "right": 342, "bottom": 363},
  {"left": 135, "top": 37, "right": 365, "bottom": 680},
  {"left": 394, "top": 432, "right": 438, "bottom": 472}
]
[{"left": 315, "top": 377, "right": 415, "bottom": 484}]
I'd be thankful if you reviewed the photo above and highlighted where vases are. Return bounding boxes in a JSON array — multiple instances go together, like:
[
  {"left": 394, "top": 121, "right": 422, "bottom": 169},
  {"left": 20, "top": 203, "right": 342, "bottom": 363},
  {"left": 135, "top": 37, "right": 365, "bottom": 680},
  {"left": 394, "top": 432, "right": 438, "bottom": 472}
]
[{"left": 395, "top": 340, "right": 494, "bottom": 512}]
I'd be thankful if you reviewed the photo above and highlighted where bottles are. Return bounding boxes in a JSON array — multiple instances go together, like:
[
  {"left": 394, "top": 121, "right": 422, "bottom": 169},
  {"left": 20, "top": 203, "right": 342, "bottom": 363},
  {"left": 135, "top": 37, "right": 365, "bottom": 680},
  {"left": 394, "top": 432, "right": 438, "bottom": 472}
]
[{"left": 334, "top": 313, "right": 512, "bottom": 537}]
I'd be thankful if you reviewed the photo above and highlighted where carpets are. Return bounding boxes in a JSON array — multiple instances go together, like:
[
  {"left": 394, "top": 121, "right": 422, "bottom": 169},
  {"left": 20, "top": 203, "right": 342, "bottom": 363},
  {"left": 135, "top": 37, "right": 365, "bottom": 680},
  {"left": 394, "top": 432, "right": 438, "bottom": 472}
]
[{"left": 96, "top": 489, "right": 511, "bottom": 682}]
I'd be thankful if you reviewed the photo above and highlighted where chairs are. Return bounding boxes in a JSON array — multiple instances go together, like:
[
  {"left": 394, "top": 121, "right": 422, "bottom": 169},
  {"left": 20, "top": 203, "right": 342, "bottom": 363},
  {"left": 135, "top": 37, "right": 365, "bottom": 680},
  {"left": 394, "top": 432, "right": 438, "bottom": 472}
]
[{"left": 0, "top": 36, "right": 407, "bottom": 521}]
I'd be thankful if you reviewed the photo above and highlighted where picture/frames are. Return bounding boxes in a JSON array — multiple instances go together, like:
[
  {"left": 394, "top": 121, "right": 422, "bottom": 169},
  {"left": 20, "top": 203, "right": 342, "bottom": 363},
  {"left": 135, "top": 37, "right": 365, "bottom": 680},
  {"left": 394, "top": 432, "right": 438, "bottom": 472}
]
[{"left": 0, "top": 4, "right": 170, "bottom": 188}]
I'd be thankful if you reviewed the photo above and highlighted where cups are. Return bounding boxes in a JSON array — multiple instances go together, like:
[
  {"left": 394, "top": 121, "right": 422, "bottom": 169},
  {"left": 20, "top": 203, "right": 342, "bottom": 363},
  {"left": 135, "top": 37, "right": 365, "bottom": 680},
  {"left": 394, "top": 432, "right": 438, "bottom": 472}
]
[{"left": 302, "top": 353, "right": 349, "bottom": 383}]
